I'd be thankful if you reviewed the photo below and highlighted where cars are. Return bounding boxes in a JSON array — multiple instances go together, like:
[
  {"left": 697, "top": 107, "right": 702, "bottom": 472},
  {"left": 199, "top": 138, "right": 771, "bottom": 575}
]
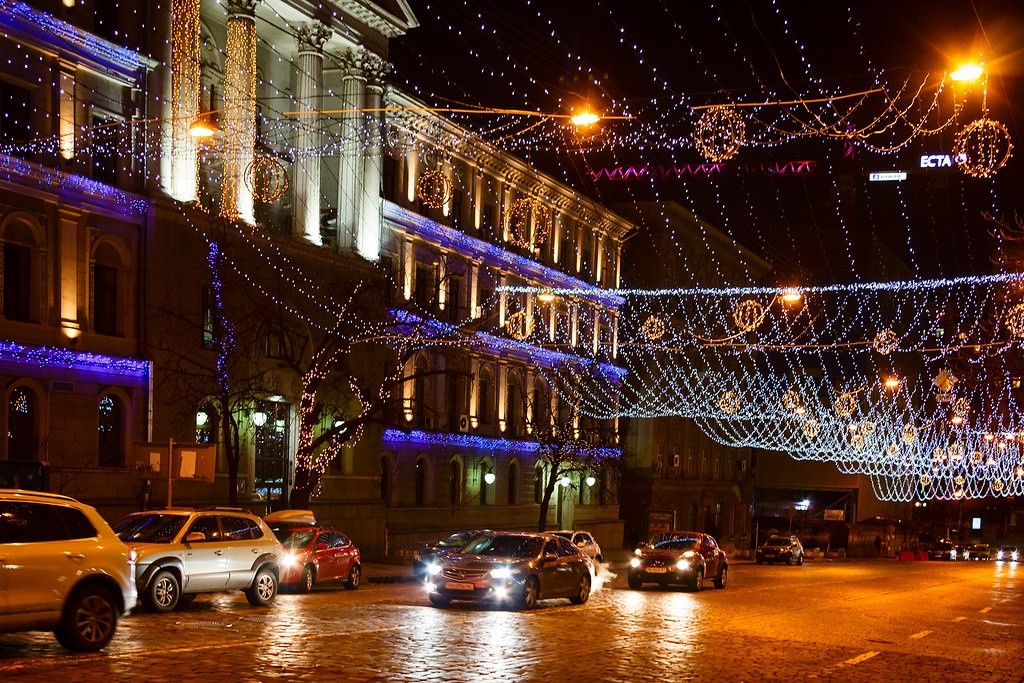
[
  {"left": 971, "top": 543, "right": 991, "bottom": 561},
  {"left": 997, "top": 544, "right": 1017, "bottom": 560},
  {"left": 272, "top": 525, "right": 360, "bottom": 592},
  {"left": 427, "top": 529, "right": 595, "bottom": 611},
  {"left": 411, "top": 529, "right": 492, "bottom": 584},
  {"left": 541, "top": 529, "right": 603, "bottom": 578},
  {"left": 950, "top": 544, "right": 970, "bottom": 561}
]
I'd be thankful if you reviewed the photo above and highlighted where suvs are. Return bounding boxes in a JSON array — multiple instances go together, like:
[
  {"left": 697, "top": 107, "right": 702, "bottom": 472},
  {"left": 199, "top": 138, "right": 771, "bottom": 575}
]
[
  {"left": 0, "top": 487, "right": 139, "bottom": 652},
  {"left": 927, "top": 537, "right": 952, "bottom": 559},
  {"left": 757, "top": 535, "right": 804, "bottom": 565},
  {"left": 107, "top": 505, "right": 285, "bottom": 611},
  {"left": 627, "top": 529, "right": 729, "bottom": 592}
]
